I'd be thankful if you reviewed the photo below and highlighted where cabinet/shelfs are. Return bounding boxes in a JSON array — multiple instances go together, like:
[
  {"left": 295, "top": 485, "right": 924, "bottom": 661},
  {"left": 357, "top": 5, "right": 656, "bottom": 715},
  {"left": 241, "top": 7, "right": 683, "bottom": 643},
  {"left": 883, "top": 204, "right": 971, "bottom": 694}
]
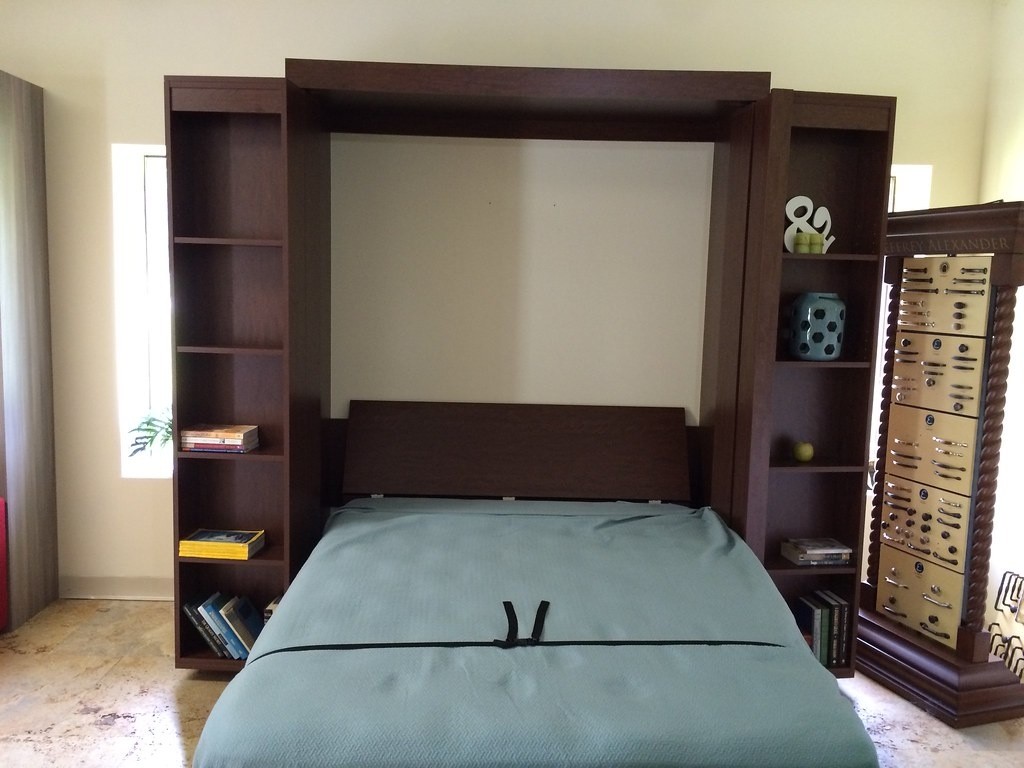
[
  {"left": 730, "top": 84, "right": 899, "bottom": 680},
  {"left": 158, "top": 74, "right": 331, "bottom": 675}
]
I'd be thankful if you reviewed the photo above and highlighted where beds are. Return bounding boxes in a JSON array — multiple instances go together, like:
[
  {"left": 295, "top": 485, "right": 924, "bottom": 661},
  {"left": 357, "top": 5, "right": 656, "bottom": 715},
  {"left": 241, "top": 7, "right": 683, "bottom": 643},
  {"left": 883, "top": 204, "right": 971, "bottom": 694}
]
[{"left": 193, "top": 491, "right": 881, "bottom": 768}]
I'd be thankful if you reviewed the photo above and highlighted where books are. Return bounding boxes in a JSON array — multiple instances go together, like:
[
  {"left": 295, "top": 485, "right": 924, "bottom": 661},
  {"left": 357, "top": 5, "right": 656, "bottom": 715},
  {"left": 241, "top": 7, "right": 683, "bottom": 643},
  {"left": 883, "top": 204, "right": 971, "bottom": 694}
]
[
  {"left": 181, "top": 442, "right": 260, "bottom": 450},
  {"left": 206, "top": 594, "right": 250, "bottom": 660},
  {"left": 798, "top": 620, "right": 812, "bottom": 656},
  {"left": 181, "top": 447, "right": 256, "bottom": 454},
  {"left": 793, "top": 534, "right": 853, "bottom": 554},
  {"left": 178, "top": 524, "right": 266, "bottom": 562},
  {"left": 262, "top": 591, "right": 284, "bottom": 631},
  {"left": 235, "top": 598, "right": 264, "bottom": 641},
  {"left": 798, "top": 595, "right": 821, "bottom": 667},
  {"left": 198, "top": 590, "right": 244, "bottom": 660},
  {"left": 182, "top": 588, "right": 230, "bottom": 658},
  {"left": 829, "top": 583, "right": 850, "bottom": 667},
  {"left": 780, "top": 550, "right": 851, "bottom": 567},
  {"left": 808, "top": 596, "right": 829, "bottom": 668},
  {"left": 191, "top": 591, "right": 238, "bottom": 659},
  {"left": 220, "top": 596, "right": 256, "bottom": 653},
  {"left": 180, "top": 420, "right": 259, "bottom": 437},
  {"left": 181, "top": 435, "right": 258, "bottom": 444},
  {"left": 782, "top": 541, "right": 849, "bottom": 560},
  {"left": 816, "top": 590, "right": 840, "bottom": 668}
]
